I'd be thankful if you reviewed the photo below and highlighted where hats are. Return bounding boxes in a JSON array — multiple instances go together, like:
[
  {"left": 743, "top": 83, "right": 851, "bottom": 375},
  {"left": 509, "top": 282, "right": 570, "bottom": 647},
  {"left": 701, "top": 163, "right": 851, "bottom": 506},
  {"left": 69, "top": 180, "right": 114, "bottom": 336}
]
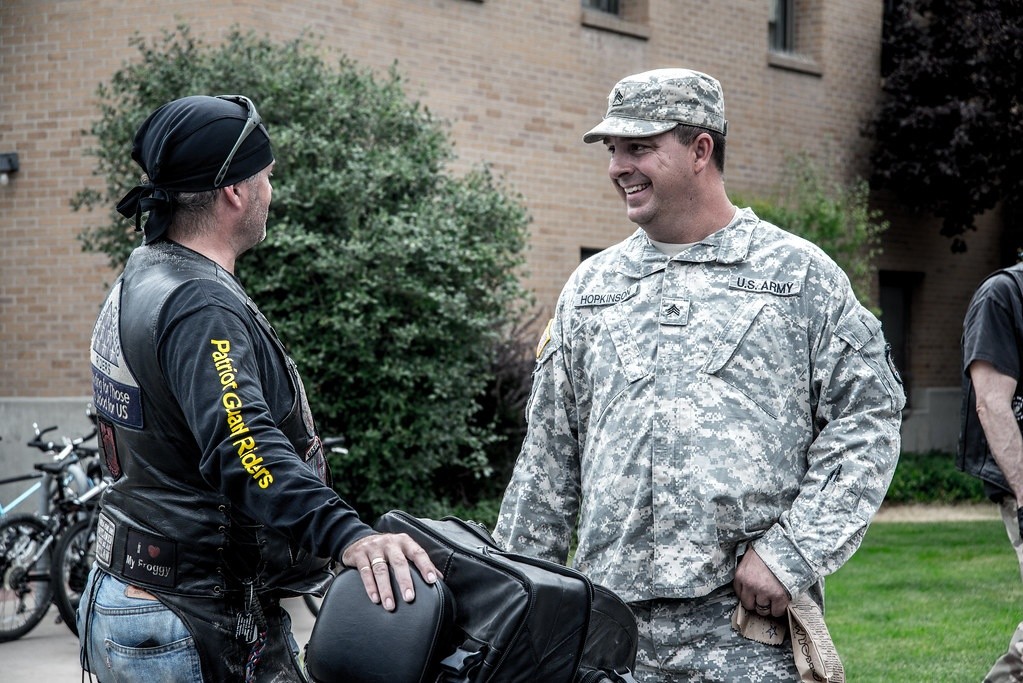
[{"left": 582, "top": 67, "right": 728, "bottom": 145}]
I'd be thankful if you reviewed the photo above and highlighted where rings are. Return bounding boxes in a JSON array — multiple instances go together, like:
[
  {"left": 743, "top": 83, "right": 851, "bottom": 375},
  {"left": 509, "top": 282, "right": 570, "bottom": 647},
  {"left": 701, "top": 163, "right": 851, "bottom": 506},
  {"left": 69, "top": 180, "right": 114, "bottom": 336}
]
[
  {"left": 361, "top": 566, "right": 370, "bottom": 570},
  {"left": 371, "top": 558, "right": 387, "bottom": 566},
  {"left": 756, "top": 604, "right": 771, "bottom": 611}
]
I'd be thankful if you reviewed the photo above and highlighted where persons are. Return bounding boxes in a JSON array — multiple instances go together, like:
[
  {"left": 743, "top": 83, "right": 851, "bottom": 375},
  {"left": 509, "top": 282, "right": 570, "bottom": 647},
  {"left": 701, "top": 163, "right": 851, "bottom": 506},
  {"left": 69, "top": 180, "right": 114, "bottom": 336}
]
[
  {"left": 961, "top": 250, "right": 1023, "bottom": 683},
  {"left": 75, "top": 93, "right": 444, "bottom": 683},
  {"left": 492, "top": 67, "right": 908, "bottom": 683}
]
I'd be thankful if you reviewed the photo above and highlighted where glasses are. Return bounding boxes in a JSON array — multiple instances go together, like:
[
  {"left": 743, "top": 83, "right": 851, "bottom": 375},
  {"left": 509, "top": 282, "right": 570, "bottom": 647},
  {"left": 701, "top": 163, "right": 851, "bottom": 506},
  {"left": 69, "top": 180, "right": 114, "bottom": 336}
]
[{"left": 214, "top": 95, "right": 262, "bottom": 187}]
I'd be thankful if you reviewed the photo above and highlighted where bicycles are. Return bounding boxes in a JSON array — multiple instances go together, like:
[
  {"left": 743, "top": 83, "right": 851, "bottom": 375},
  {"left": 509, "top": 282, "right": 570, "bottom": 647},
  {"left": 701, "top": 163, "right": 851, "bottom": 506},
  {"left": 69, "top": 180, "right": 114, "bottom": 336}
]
[
  {"left": 301, "top": 436, "right": 349, "bottom": 618},
  {"left": 0, "top": 410, "right": 108, "bottom": 643}
]
